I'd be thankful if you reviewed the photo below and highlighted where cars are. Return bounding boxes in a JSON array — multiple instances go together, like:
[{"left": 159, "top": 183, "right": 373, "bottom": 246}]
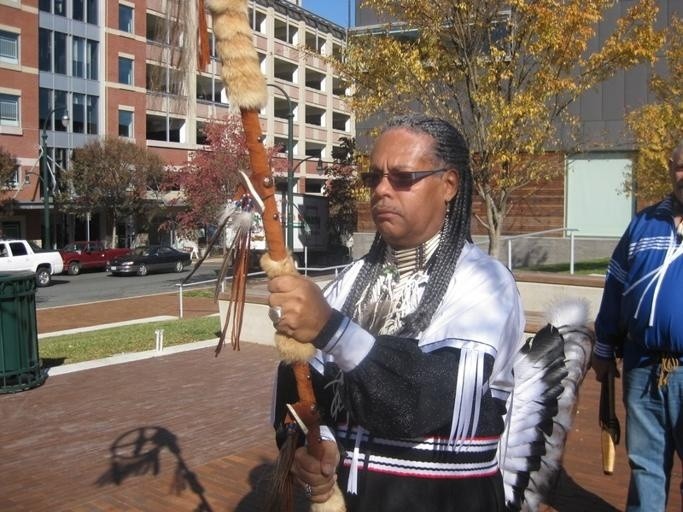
[{"left": 105, "top": 244, "right": 192, "bottom": 277}]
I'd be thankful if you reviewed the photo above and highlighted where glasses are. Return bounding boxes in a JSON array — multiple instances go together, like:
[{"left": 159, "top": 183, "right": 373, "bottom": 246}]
[{"left": 358, "top": 167, "right": 448, "bottom": 190}]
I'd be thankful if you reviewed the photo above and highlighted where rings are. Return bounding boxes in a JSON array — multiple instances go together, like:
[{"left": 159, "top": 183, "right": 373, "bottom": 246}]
[
  {"left": 273, "top": 305, "right": 281, "bottom": 317},
  {"left": 302, "top": 483, "right": 312, "bottom": 498}
]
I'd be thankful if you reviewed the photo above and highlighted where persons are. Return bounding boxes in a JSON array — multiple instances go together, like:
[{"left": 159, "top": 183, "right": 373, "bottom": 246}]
[
  {"left": 589, "top": 142, "right": 683, "bottom": 511},
  {"left": 263, "top": 113, "right": 529, "bottom": 511}
]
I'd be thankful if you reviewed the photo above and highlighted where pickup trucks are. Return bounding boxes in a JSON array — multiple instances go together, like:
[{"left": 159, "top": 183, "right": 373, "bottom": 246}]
[
  {"left": 58, "top": 239, "right": 132, "bottom": 276},
  {"left": 0, "top": 238, "right": 64, "bottom": 287}
]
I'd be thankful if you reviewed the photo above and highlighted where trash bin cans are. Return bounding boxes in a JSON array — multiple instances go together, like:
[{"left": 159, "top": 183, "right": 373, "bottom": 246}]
[{"left": 0, "top": 271, "right": 49, "bottom": 392}]
